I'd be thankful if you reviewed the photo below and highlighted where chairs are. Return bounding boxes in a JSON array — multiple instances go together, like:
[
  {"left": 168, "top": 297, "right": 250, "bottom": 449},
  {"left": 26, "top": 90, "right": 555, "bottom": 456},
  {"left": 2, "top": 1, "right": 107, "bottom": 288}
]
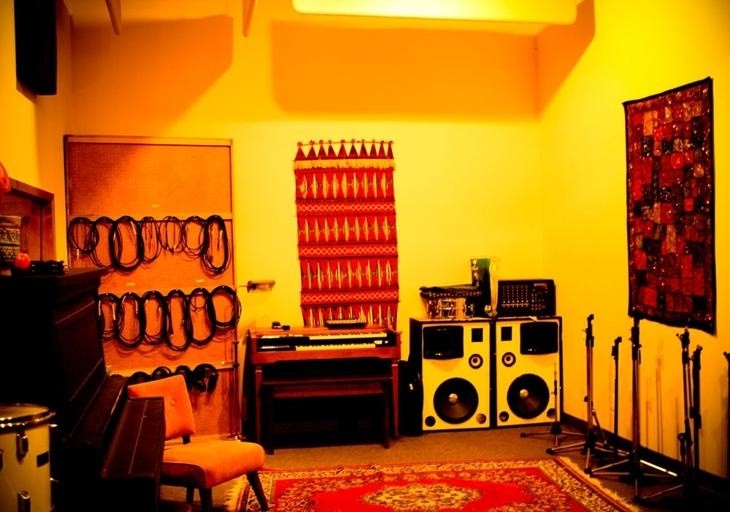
[{"left": 127, "top": 373, "right": 269, "bottom": 511}]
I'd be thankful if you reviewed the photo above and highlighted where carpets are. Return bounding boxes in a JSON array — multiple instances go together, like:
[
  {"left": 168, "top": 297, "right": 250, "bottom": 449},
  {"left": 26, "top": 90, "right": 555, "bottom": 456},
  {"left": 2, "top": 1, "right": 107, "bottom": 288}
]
[{"left": 226, "top": 451, "right": 645, "bottom": 512}]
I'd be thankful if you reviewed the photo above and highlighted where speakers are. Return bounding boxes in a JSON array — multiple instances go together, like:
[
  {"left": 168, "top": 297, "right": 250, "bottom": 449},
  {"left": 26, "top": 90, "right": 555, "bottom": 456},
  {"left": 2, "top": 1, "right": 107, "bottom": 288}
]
[
  {"left": 408, "top": 318, "right": 492, "bottom": 431},
  {"left": 492, "top": 316, "right": 564, "bottom": 427},
  {"left": 14, "top": 0, "right": 58, "bottom": 95}
]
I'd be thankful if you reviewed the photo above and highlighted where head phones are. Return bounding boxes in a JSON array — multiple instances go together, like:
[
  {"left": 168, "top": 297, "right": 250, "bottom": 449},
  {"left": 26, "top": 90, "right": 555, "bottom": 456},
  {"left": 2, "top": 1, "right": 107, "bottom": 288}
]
[{"left": 130, "top": 362, "right": 219, "bottom": 392}]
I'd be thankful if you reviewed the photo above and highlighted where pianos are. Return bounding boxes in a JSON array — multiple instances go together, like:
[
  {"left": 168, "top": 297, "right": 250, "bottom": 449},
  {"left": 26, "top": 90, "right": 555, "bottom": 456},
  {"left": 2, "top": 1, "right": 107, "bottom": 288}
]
[
  {"left": 1, "top": 260, "right": 166, "bottom": 511},
  {"left": 247, "top": 326, "right": 401, "bottom": 449}
]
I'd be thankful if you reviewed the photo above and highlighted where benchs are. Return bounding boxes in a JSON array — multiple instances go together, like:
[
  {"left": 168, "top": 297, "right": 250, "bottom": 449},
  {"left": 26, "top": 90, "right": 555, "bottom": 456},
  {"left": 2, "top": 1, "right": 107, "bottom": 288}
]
[{"left": 270, "top": 382, "right": 390, "bottom": 450}]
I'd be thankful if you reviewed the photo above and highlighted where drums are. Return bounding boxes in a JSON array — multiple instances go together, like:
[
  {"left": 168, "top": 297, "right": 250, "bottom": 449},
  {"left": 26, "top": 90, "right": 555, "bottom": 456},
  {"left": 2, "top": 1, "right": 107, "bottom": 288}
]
[{"left": 0, "top": 403, "right": 59, "bottom": 510}]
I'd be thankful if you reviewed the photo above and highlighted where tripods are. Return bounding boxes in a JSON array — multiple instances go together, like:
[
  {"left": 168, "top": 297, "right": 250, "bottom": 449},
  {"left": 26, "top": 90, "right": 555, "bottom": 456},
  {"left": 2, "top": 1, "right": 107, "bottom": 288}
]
[{"left": 520, "top": 305, "right": 730, "bottom": 512}]
